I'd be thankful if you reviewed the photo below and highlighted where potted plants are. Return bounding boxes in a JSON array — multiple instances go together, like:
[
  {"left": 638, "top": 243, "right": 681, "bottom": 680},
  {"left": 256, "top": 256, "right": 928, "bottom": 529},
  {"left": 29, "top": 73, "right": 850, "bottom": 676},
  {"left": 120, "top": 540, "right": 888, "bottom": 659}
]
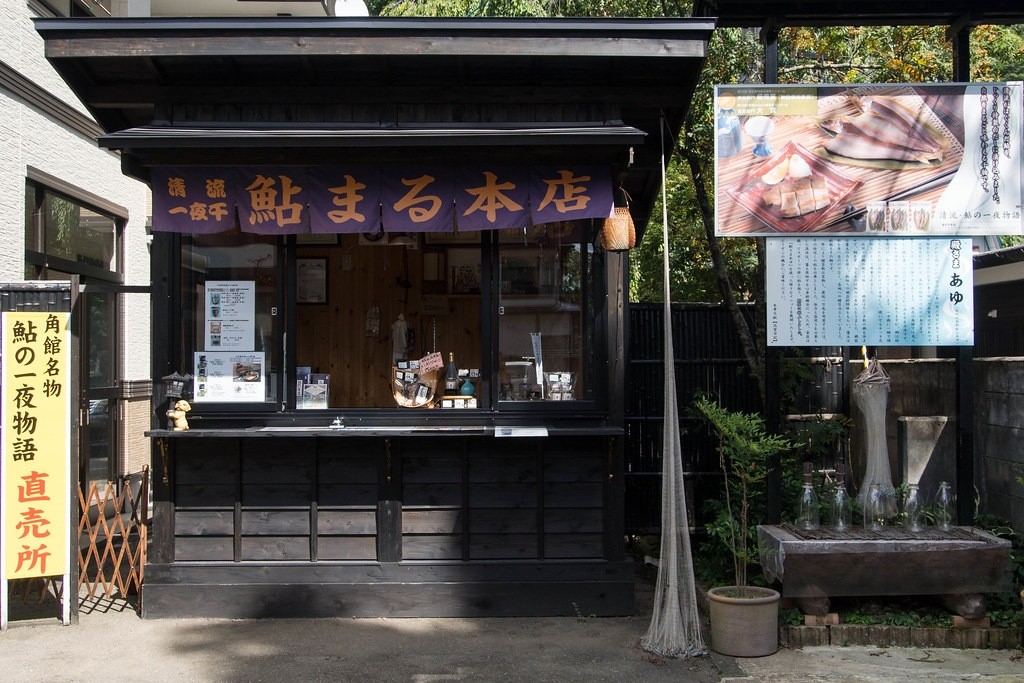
[{"left": 693, "top": 395, "right": 818, "bottom": 658}]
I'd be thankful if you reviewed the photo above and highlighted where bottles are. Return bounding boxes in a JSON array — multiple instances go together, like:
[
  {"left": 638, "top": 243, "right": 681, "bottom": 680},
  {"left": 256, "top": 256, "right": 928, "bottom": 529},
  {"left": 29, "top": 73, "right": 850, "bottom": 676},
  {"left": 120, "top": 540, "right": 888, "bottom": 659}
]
[
  {"left": 445, "top": 353, "right": 461, "bottom": 395},
  {"left": 903, "top": 484, "right": 928, "bottom": 533},
  {"left": 866, "top": 483, "right": 890, "bottom": 531},
  {"left": 798, "top": 482, "right": 819, "bottom": 529},
  {"left": 934, "top": 482, "right": 957, "bottom": 532},
  {"left": 831, "top": 482, "right": 852, "bottom": 532}
]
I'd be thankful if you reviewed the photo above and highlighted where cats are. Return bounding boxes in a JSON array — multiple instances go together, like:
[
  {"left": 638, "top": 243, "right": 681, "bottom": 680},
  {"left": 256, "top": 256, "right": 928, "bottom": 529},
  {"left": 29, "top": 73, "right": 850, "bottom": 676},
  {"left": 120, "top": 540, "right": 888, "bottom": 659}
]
[{"left": 165, "top": 399, "right": 192, "bottom": 432}]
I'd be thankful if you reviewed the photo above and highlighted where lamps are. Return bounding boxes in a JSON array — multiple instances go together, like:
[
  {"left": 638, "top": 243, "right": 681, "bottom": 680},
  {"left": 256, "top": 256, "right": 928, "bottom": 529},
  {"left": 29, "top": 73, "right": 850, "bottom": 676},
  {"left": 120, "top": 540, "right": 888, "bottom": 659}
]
[{"left": 599, "top": 171, "right": 636, "bottom": 253}]
